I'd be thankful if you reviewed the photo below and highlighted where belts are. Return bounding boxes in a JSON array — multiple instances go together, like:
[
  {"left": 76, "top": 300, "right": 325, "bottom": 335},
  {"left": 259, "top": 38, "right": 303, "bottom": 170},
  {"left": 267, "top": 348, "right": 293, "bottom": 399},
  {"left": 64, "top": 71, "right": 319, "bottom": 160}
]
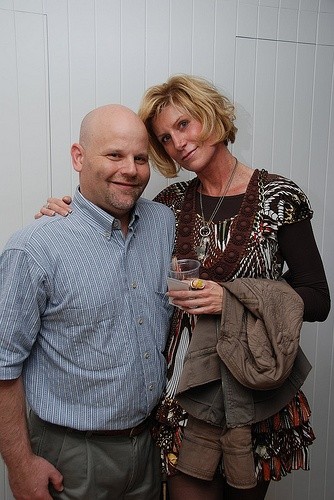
[{"left": 34, "top": 412, "right": 150, "bottom": 440}]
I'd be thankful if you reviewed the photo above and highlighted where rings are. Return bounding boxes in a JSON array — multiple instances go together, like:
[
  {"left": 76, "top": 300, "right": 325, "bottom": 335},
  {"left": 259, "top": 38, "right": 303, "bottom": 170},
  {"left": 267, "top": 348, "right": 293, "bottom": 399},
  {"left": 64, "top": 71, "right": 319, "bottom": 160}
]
[
  {"left": 190, "top": 279, "right": 206, "bottom": 290},
  {"left": 43, "top": 203, "right": 50, "bottom": 209}
]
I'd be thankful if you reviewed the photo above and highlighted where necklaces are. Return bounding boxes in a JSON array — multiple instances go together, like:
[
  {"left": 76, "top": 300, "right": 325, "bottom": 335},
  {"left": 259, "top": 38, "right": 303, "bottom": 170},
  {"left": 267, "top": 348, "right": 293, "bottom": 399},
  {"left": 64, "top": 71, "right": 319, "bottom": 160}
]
[{"left": 196, "top": 157, "right": 237, "bottom": 261}]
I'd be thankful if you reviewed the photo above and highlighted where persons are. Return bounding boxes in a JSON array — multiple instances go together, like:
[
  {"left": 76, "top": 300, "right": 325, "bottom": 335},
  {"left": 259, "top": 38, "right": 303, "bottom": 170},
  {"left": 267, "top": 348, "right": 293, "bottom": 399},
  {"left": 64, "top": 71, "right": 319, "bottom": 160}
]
[
  {"left": 32, "top": 73, "right": 331, "bottom": 500},
  {"left": 0, "top": 102, "right": 178, "bottom": 500}
]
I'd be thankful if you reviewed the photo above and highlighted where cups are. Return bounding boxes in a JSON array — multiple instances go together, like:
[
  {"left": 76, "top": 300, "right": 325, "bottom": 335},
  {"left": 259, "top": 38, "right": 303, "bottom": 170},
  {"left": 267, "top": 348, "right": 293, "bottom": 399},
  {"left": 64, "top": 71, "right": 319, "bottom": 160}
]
[{"left": 167, "top": 259, "right": 201, "bottom": 308}]
